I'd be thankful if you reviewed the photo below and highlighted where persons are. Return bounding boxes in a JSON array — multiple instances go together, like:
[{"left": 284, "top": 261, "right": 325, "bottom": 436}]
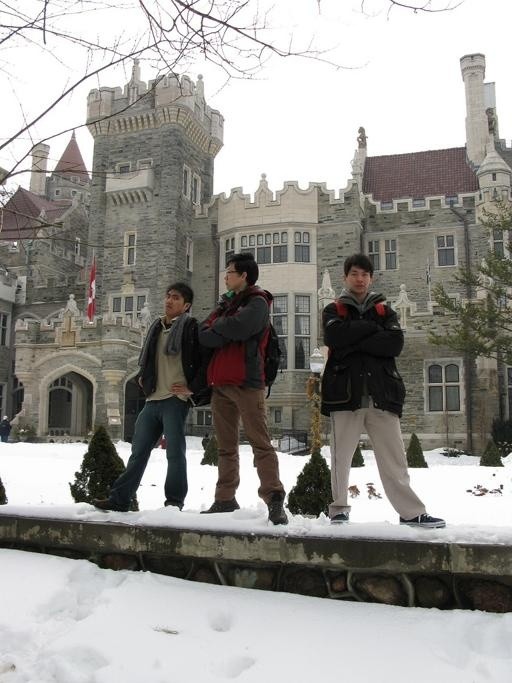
[
  {"left": 195, "top": 251, "right": 289, "bottom": 525},
  {"left": 1, "top": 415, "right": 11, "bottom": 441},
  {"left": 88, "top": 281, "right": 211, "bottom": 514},
  {"left": 315, "top": 251, "right": 450, "bottom": 529}
]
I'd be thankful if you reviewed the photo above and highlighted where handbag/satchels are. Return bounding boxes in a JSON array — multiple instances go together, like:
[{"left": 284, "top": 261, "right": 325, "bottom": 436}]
[{"left": 264, "top": 323, "right": 282, "bottom": 387}]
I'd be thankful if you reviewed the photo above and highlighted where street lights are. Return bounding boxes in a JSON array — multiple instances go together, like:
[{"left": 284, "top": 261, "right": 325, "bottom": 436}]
[{"left": 308, "top": 347, "right": 325, "bottom": 454}]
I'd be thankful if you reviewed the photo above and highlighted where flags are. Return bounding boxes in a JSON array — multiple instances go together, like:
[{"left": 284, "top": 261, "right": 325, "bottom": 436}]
[{"left": 86, "top": 256, "right": 99, "bottom": 321}]
